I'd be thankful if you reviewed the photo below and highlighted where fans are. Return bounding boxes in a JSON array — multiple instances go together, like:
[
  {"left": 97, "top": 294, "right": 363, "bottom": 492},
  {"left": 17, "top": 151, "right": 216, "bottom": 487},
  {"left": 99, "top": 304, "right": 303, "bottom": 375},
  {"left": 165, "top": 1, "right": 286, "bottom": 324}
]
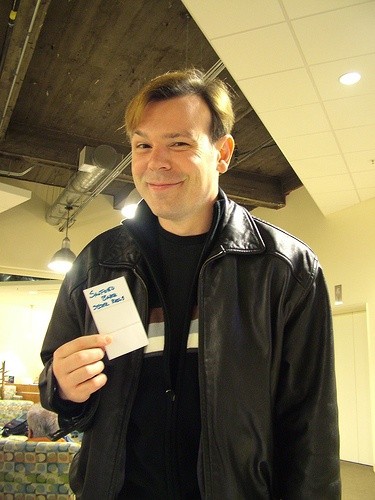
[{"left": 49, "top": 207, "right": 76, "bottom": 273}]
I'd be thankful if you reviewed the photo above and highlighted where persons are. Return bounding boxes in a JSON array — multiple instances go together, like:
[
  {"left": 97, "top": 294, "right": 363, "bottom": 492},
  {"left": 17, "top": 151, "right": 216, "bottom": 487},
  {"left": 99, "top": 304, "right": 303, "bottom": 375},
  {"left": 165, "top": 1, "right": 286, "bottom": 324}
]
[
  {"left": 26, "top": 402, "right": 74, "bottom": 444},
  {"left": 37, "top": 70, "right": 341, "bottom": 500}
]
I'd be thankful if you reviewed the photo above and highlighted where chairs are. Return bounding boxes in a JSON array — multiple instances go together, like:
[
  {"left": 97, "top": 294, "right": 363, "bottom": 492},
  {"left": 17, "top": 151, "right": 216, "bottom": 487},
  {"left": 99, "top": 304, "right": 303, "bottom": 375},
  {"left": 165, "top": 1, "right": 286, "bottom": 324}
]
[
  {"left": 0, "top": 400, "right": 34, "bottom": 437},
  {"left": 0, "top": 438, "right": 82, "bottom": 500}
]
